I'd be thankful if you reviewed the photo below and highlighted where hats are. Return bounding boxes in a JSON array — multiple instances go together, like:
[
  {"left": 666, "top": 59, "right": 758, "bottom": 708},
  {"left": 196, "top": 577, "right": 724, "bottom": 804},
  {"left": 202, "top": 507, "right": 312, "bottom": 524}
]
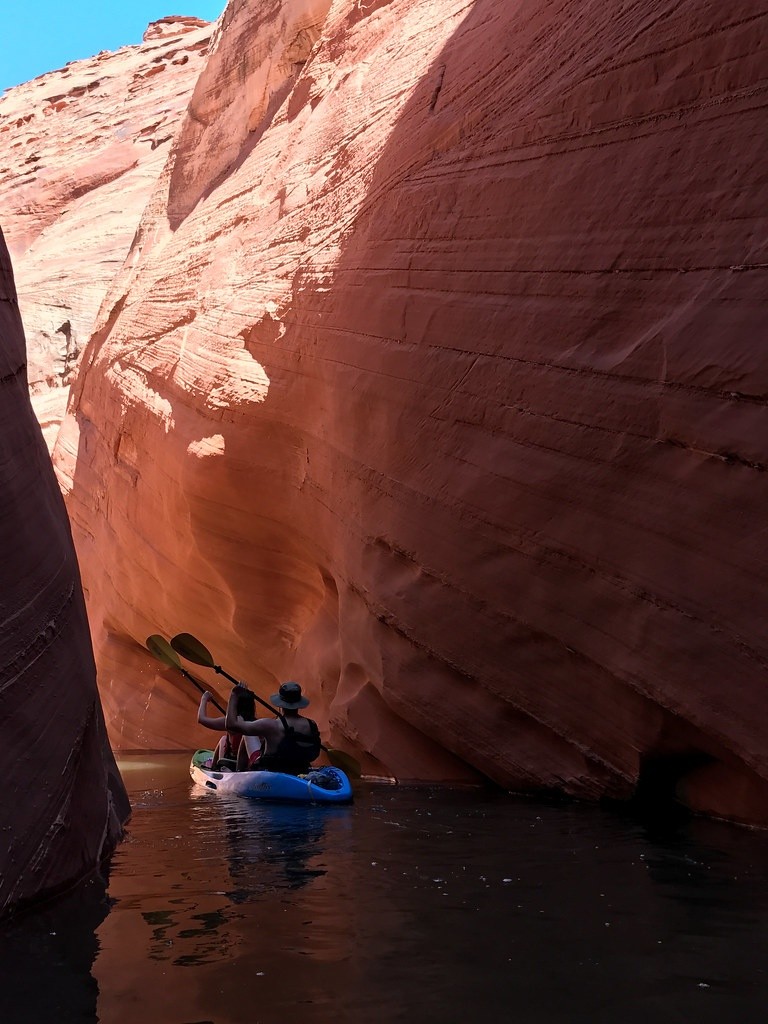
[{"left": 270, "top": 682, "right": 310, "bottom": 709}]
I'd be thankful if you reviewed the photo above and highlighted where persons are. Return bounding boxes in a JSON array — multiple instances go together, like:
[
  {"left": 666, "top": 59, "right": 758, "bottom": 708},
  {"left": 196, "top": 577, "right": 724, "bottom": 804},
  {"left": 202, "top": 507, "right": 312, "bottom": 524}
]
[
  {"left": 196, "top": 681, "right": 264, "bottom": 771},
  {"left": 224, "top": 681, "right": 320, "bottom": 776}
]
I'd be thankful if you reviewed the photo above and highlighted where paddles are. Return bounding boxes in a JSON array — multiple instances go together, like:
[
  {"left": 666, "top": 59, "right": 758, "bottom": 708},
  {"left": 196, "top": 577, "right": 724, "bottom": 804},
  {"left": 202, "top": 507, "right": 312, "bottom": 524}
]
[
  {"left": 169, "top": 630, "right": 362, "bottom": 783},
  {"left": 145, "top": 633, "right": 228, "bottom": 714}
]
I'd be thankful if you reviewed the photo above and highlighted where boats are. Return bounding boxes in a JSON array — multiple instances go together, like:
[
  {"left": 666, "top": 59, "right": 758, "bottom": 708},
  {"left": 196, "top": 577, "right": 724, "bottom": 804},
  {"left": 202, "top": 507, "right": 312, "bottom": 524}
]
[{"left": 190, "top": 746, "right": 351, "bottom": 806}]
